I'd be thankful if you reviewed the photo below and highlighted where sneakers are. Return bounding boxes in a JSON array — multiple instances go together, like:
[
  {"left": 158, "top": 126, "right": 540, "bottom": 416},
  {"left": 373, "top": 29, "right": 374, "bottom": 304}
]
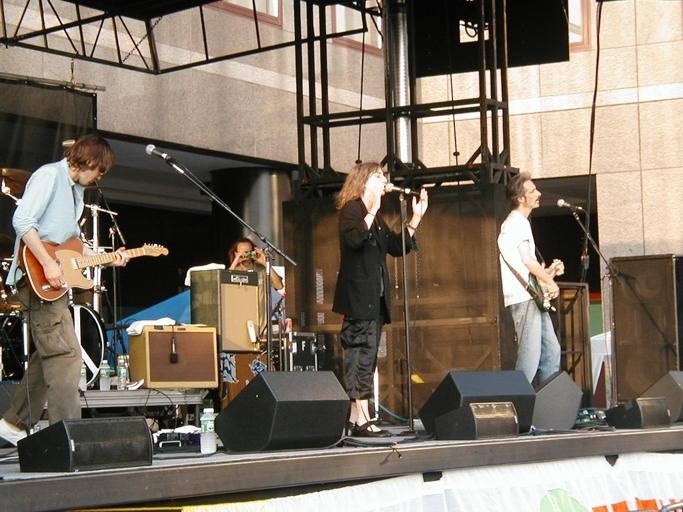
[{"left": 0, "top": 418, "right": 28, "bottom": 448}]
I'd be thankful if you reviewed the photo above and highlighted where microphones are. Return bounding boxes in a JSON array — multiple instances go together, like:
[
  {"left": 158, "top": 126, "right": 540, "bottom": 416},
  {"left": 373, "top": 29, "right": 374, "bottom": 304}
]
[
  {"left": 556, "top": 198, "right": 582, "bottom": 211},
  {"left": 384, "top": 183, "right": 420, "bottom": 195},
  {"left": 169, "top": 337, "right": 178, "bottom": 364},
  {"left": 145, "top": 144, "right": 174, "bottom": 161}
]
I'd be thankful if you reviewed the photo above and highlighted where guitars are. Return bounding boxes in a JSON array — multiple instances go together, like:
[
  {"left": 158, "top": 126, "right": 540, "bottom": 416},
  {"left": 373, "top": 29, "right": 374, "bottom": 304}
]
[
  {"left": 17, "top": 235, "right": 170, "bottom": 303},
  {"left": 526, "top": 258, "right": 566, "bottom": 311}
]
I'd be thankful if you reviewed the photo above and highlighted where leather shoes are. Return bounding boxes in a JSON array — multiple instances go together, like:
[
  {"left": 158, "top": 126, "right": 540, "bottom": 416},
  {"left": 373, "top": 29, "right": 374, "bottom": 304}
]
[
  {"left": 345, "top": 421, "right": 356, "bottom": 432},
  {"left": 352, "top": 420, "right": 392, "bottom": 437}
]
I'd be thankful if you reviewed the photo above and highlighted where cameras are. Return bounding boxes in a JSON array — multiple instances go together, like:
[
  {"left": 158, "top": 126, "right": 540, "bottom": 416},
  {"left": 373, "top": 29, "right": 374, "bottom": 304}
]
[{"left": 244, "top": 251, "right": 257, "bottom": 258}]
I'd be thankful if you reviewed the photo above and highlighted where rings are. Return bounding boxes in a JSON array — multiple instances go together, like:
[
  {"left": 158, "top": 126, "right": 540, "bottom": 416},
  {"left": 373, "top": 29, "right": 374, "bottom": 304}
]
[{"left": 125, "top": 259, "right": 129, "bottom": 262}]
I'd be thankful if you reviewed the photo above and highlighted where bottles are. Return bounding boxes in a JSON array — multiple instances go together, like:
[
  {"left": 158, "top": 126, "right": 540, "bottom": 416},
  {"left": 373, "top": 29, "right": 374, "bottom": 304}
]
[
  {"left": 199, "top": 409, "right": 215, "bottom": 455},
  {"left": 97, "top": 359, "right": 109, "bottom": 390},
  {"left": 79, "top": 360, "right": 86, "bottom": 391},
  {"left": 115, "top": 355, "right": 126, "bottom": 391}
]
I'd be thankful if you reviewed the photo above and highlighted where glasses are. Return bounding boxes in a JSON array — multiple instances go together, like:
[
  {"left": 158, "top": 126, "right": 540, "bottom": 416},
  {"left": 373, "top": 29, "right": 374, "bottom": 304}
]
[{"left": 94, "top": 159, "right": 108, "bottom": 177}]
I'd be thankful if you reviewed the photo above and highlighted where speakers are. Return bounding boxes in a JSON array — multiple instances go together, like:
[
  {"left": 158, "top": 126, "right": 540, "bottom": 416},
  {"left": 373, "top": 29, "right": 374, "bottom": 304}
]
[
  {"left": 190, "top": 269, "right": 259, "bottom": 351},
  {"left": 605, "top": 397, "right": 671, "bottom": 429},
  {"left": 17, "top": 416, "right": 153, "bottom": 472},
  {"left": 434, "top": 400, "right": 521, "bottom": 440}
]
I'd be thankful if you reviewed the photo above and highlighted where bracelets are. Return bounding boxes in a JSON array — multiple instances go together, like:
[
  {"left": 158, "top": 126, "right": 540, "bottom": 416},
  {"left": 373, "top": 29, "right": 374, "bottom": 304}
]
[{"left": 407, "top": 223, "right": 417, "bottom": 232}]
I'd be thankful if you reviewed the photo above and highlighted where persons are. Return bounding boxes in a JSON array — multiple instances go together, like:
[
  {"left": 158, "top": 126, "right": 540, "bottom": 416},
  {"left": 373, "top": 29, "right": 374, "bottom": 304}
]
[
  {"left": 496, "top": 174, "right": 565, "bottom": 386},
  {"left": 334, "top": 163, "right": 428, "bottom": 438},
  {"left": 0, "top": 135, "right": 129, "bottom": 449},
  {"left": 226, "top": 240, "right": 283, "bottom": 292}
]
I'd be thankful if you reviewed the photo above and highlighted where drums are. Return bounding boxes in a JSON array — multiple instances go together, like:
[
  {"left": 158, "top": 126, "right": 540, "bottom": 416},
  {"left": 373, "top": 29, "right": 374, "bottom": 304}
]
[{"left": 1, "top": 304, "right": 109, "bottom": 387}]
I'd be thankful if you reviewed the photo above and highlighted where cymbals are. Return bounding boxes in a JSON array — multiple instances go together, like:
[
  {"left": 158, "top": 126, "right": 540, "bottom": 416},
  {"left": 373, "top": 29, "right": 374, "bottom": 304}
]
[{"left": 1, "top": 167, "right": 34, "bottom": 196}]
